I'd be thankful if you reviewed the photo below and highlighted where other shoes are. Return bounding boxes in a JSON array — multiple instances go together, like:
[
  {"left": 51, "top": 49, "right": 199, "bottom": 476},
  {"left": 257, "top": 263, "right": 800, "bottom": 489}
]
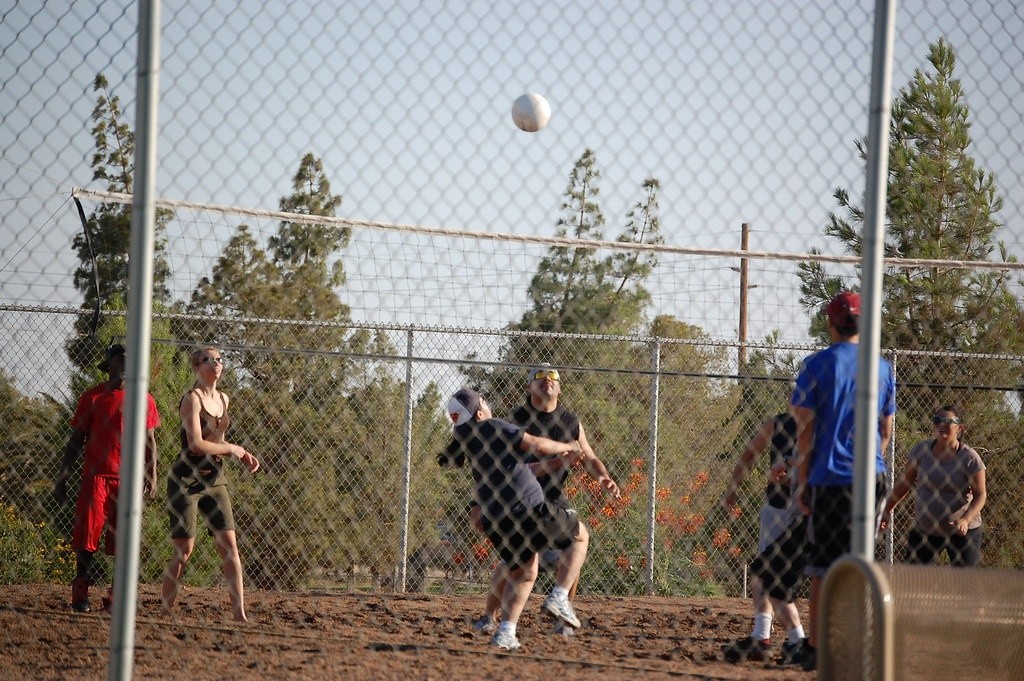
[
  {"left": 802, "top": 646, "right": 819, "bottom": 672},
  {"left": 70, "top": 577, "right": 91, "bottom": 612},
  {"left": 476, "top": 615, "right": 495, "bottom": 632},
  {"left": 778, "top": 637, "right": 807, "bottom": 665},
  {"left": 725, "top": 636, "right": 773, "bottom": 661},
  {"left": 555, "top": 624, "right": 575, "bottom": 637}
]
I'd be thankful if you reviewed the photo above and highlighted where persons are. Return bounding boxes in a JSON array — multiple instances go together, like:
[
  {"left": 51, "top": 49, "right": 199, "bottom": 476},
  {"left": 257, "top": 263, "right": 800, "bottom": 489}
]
[
  {"left": 725, "top": 413, "right": 806, "bottom": 665},
  {"left": 879, "top": 406, "right": 986, "bottom": 568},
  {"left": 725, "top": 291, "right": 898, "bottom": 670},
  {"left": 437, "top": 363, "right": 620, "bottom": 650},
  {"left": 164, "top": 347, "right": 260, "bottom": 627},
  {"left": 54, "top": 344, "right": 161, "bottom": 611},
  {"left": 436, "top": 387, "right": 589, "bottom": 651}
]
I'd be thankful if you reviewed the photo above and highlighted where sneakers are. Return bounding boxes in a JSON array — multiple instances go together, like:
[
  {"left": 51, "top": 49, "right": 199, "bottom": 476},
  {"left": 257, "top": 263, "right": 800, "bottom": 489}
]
[
  {"left": 491, "top": 630, "right": 521, "bottom": 650},
  {"left": 540, "top": 593, "right": 581, "bottom": 628}
]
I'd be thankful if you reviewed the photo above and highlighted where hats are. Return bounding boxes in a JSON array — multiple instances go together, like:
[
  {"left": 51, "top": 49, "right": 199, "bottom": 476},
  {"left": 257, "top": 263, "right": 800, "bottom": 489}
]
[
  {"left": 97, "top": 342, "right": 126, "bottom": 371},
  {"left": 447, "top": 387, "right": 480, "bottom": 434},
  {"left": 527, "top": 362, "right": 561, "bottom": 382},
  {"left": 823, "top": 290, "right": 862, "bottom": 327}
]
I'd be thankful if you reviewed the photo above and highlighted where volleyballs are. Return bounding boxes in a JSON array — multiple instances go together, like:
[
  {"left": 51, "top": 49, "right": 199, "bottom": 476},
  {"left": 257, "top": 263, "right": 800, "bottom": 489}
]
[{"left": 512, "top": 94, "right": 551, "bottom": 132}]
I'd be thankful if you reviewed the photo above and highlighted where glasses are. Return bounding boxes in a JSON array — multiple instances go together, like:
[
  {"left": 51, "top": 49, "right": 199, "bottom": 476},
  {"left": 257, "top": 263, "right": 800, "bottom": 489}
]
[
  {"left": 199, "top": 356, "right": 223, "bottom": 364},
  {"left": 933, "top": 416, "right": 959, "bottom": 425},
  {"left": 530, "top": 371, "right": 559, "bottom": 381}
]
